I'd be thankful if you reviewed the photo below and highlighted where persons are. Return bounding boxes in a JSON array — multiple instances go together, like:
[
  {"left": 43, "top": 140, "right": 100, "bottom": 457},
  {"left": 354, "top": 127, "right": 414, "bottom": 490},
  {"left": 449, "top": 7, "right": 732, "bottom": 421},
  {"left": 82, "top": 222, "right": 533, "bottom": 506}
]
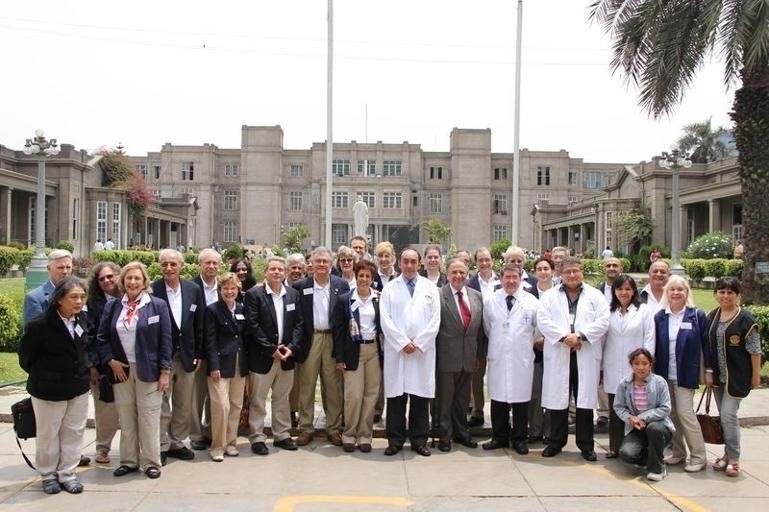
[
  {"left": 351, "top": 195, "right": 368, "bottom": 238},
  {"left": 19, "top": 236, "right": 763, "bottom": 492}
]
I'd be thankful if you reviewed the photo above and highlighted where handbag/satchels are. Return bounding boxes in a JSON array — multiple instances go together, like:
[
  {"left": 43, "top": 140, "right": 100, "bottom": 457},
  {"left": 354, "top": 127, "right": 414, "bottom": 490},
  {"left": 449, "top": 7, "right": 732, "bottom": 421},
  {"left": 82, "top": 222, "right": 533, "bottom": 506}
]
[
  {"left": 12, "top": 398, "right": 36, "bottom": 438},
  {"left": 696, "top": 414, "right": 724, "bottom": 444}
]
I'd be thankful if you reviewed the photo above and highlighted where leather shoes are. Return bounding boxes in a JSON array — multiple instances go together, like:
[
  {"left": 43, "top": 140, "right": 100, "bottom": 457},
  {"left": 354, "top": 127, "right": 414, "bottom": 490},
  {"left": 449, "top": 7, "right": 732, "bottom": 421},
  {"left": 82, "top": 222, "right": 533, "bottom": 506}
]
[
  {"left": 582, "top": 450, "right": 598, "bottom": 461},
  {"left": 529, "top": 436, "right": 561, "bottom": 456},
  {"left": 297, "top": 431, "right": 528, "bottom": 455},
  {"left": 467, "top": 415, "right": 484, "bottom": 427},
  {"left": 274, "top": 438, "right": 297, "bottom": 450},
  {"left": 597, "top": 416, "right": 608, "bottom": 424},
  {"left": 252, "top": 442, "right": 269, "bottom": 454},
  {"left": 160, "top": 427, "right": 239, "bottom": 466}
]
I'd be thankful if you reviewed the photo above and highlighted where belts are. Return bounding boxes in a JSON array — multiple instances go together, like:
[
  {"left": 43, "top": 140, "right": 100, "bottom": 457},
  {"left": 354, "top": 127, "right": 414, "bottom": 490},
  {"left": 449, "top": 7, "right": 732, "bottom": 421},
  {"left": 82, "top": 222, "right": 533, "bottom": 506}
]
[
  {"left": 360, "top": 340, "right": 375, "bottom": 344},
  {"left": 315, "top": 329, "right": 332, "bottom": 334}
]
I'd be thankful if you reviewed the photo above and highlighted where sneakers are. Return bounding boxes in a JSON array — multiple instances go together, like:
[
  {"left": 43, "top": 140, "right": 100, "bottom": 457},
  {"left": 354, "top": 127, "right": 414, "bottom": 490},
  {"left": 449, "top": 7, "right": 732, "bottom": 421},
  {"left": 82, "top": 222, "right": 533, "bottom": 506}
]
[
  {"left": 606, "top": 451, "right": 617, "bottom": 457},
  {"left": 62, "top": 480, "right": 84, "bottom": 493},
  {"left": 114, "top": 465, "right": 138, "bottom": 475},
  {"left": 634, "top": 456, "right": 739, "bottom": 481},
  {"left": 79, "top": 455, "right": 91, "bottom": 466},
  {"left": 146, "top": 466, "right": 161, "bottom": 478},
  {"left": 43, "top": 479, "right": 61, "bottom": 494},
  {"left": 96, "top": 452, "right": 110, "bottom": 462}
]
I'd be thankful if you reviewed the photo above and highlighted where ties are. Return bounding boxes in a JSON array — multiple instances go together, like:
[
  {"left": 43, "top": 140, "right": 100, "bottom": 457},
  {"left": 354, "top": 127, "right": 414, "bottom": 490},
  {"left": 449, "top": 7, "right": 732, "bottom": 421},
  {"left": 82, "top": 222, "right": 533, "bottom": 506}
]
[
  {"left": 506, "top": 295, "right": 514, "bottom": 311},
  {"left": 409, "top": 280, "right": 414, "bottom": 297},
  {"left": 455, "top": 291, "right": 471, "bottom": 330}
]
